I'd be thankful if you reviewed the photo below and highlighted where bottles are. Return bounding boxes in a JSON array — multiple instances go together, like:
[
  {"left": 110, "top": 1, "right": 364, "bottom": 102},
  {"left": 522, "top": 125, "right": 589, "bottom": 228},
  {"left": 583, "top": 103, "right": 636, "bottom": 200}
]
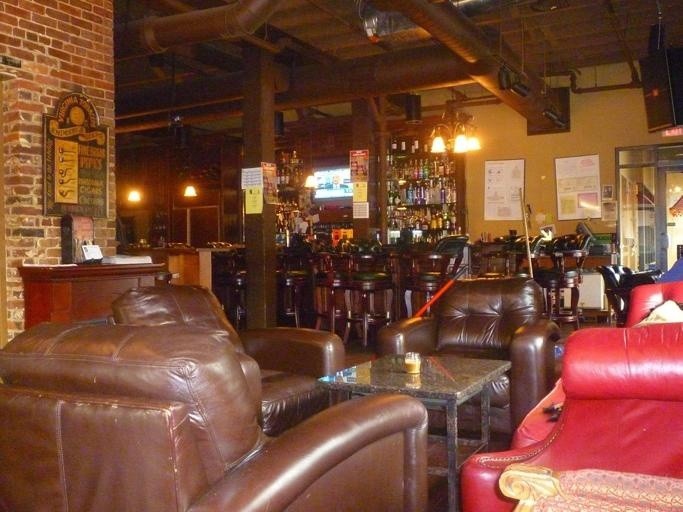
[
  {"left": 274, "top": 148, "right": 309, "bottom": 248},
  {"left": 404, "top": 352, "right": 422, "bottom": 374},
  {"left": 386, "top": 135, "right": 462, "bottom": 247}
]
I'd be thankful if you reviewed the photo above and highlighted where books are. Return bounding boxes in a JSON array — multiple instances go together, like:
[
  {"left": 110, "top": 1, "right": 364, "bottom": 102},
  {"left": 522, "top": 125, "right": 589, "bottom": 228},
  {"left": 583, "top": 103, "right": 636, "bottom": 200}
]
[{"left": 102, "top": 255, "right": 152, "bottom": 265}]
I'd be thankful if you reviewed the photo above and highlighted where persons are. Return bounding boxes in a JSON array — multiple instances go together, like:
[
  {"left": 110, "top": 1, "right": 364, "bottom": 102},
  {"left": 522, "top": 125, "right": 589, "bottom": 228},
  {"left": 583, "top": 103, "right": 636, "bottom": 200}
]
[{"left": 324, "top": 171, "right": 348, "bottom": 191}]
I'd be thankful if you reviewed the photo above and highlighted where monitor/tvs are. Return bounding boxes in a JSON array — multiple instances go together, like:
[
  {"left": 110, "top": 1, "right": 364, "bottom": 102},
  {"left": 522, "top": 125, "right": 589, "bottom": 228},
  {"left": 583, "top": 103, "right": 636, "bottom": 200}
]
[{"left": 312, "top": 165, "right": 352, "bottom": 205}]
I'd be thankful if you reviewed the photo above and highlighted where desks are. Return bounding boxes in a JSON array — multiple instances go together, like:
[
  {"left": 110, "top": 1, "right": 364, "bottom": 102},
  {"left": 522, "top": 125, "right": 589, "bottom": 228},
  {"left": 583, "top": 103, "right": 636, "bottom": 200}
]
[{"left": 316, "top": 353, "right": 513, "bottom": 511}]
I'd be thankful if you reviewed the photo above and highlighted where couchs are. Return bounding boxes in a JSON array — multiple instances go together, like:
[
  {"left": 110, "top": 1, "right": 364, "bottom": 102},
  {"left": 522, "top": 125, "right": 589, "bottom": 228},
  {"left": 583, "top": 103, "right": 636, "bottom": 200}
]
[
  {"left": 84, "top": 284, "right": 345, "bottom": 437},
  {"left": 459, "top": 282, "right": 683, "bottom": 511},
  {"left": 374, "top": 274, "right": 562, "bottom": 438},
  {"left": 0, "top": 322, "right": 428, "bottom": 512}
]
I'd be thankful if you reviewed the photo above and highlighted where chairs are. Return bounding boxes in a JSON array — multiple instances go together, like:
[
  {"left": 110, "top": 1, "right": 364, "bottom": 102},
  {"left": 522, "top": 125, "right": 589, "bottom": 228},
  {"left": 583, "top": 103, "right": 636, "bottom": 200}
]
[
  {"left": 374, "top": 274, "right": 562, "bottom": 438},
  {"left": 210, "top": 245, "right": 661, "bottom": 354}
]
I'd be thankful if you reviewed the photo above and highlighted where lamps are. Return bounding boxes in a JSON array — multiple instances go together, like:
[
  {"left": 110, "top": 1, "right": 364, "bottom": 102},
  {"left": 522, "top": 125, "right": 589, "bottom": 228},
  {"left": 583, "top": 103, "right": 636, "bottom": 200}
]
[
  {"left": 497, "top": 0, "right": 569, "bottom": 128},
  {"left": 404, "top": 90, "right": 424, "bottom": 126},
  {"left": 273, "top": 110, "right": 285, "bottom": 138},
  {"left": 426, "top": 89, "right": 482, "bottom": 155}
]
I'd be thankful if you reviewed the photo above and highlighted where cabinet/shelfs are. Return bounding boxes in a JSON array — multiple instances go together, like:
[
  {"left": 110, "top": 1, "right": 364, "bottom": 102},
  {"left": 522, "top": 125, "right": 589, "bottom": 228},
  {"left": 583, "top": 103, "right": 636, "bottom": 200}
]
[{"left": 377, "top": 152, "right": 466, "bottom": 245}]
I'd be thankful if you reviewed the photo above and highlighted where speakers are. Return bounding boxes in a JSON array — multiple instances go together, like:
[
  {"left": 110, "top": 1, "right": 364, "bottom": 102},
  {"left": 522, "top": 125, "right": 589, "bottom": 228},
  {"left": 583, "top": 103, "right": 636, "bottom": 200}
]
[{"left": 639, "top": 44, "right": 683, "bottom": 133}]
